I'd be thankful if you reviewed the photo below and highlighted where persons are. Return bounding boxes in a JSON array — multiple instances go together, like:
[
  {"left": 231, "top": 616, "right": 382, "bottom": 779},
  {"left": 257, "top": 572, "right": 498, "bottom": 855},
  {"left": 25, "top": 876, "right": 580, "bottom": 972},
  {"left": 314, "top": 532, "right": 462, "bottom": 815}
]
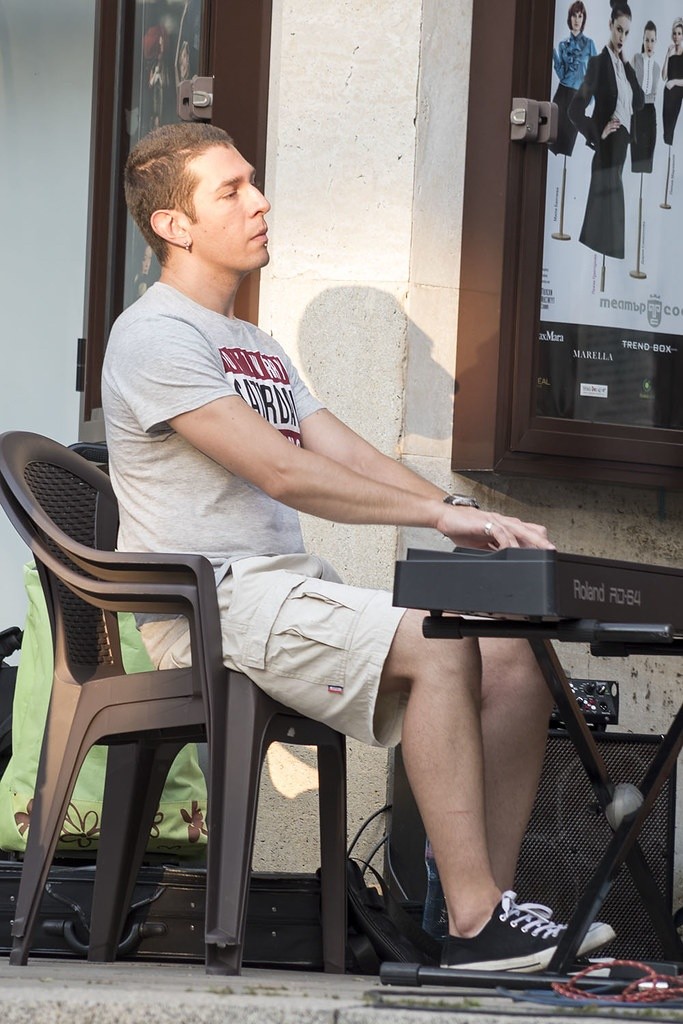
[
  {"left": 100, "top": 121, "right": 618, "bottom": 971},
  {"left": 630, "top": 20, "right": 660, "bottom": 172},
  {"left": 661, "top": 15, "right": 683, "bottom": 144},
  {"left": 177, "top": 41, "right": 190, "bottom": 81},
  {"left": 548, "top": 0, "right": 597, "bottom": 156},
  {"left": 147, "top": 28, "right": 170, "bottom": 127},
  {"left": 566, "top": 0, "right": 645, "bottom": 258}
]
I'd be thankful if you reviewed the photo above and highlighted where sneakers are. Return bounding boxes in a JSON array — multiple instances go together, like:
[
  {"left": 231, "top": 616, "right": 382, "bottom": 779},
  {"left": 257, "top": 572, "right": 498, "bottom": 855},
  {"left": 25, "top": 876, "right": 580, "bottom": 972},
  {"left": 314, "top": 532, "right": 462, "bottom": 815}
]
[{"left": 440, "top": 890, "right": 617, "bottom": 972}]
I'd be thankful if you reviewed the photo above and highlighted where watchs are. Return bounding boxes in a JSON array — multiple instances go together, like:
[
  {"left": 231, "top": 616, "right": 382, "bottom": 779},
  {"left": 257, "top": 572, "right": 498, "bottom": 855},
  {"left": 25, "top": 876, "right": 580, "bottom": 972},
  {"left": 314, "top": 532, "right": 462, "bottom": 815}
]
[{"left": 443, "top": 493, "right": 480, "bottom": 508}]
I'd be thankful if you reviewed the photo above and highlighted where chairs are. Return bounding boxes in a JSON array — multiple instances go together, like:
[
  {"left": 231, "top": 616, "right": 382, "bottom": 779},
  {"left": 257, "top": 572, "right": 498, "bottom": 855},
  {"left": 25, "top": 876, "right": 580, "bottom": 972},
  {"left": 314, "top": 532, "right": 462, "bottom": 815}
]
[{"left": 0, "top": 427, "right": 347, "bottom": 979}]
